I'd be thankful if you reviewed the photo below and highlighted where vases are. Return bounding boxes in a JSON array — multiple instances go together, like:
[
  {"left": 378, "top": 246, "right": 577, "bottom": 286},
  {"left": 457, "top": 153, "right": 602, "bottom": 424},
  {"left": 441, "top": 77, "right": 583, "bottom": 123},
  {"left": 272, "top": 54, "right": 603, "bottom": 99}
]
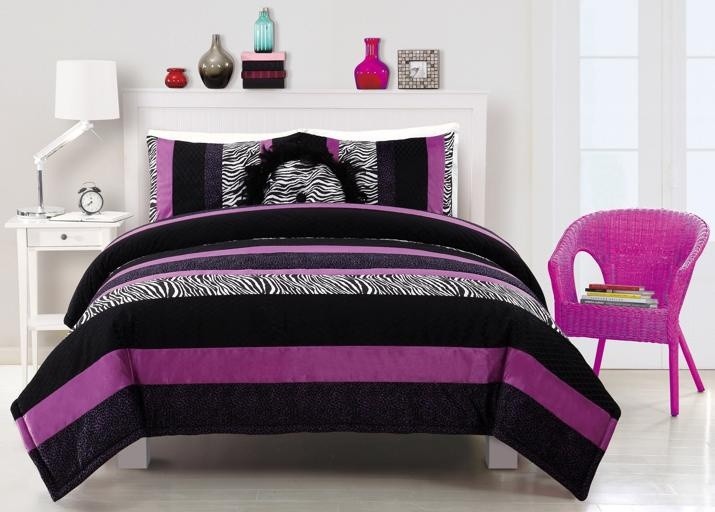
[
  {"left": 199, "top": 34, "right": 232, "bottom": 89},
  {"left": 353, "top": 37, "right": 389, "bottom": 89}
]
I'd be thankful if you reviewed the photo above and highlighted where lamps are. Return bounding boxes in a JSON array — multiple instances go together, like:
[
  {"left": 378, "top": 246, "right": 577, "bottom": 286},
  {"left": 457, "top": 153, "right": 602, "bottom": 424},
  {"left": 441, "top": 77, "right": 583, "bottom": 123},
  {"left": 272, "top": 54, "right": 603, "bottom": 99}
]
[{"left": 17, "top": 57, "right": 127, "bottom": 215}]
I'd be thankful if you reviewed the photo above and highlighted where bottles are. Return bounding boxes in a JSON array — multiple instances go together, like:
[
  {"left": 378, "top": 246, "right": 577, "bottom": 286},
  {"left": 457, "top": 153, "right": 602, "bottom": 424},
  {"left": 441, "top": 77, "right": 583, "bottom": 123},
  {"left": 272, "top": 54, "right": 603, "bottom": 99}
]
[{"left": 253, "top": 8, "right": 274, "bottom": 53}]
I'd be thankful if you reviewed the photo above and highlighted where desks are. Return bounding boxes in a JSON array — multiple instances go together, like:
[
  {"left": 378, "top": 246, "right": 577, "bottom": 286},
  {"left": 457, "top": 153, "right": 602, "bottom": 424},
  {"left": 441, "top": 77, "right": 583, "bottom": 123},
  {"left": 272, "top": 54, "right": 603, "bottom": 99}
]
[{"left": 6, "top": 215, "right": 131, "bottom": 382}]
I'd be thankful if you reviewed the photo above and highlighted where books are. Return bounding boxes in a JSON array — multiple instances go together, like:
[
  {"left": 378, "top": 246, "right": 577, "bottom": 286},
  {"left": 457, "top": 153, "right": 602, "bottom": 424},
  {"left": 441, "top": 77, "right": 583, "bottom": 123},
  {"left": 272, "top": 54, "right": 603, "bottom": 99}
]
[
  {"left": 579, "top": 283, "right": 659, "bottom": 309},
  {"left": 50, "top": 211, "right": 134, "bottom": 223}
]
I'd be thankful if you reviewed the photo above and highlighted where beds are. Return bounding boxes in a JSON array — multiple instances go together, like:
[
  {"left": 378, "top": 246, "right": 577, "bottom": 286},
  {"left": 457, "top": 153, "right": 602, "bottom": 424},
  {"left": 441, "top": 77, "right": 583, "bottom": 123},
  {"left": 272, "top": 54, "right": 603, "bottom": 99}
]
[{"left": 10, "top": 87, "right": 621, "bottom": 504}]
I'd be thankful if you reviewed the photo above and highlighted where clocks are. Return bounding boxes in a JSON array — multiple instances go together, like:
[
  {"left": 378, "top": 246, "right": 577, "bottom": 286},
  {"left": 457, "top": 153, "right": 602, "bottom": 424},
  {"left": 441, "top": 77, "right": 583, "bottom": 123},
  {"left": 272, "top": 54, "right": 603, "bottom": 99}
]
[
  {"left": 398, "top": 49, "right": 439, "bottom": 89},
  {"left": 77, "top": 181, "right": 104, "bottom": 216}
]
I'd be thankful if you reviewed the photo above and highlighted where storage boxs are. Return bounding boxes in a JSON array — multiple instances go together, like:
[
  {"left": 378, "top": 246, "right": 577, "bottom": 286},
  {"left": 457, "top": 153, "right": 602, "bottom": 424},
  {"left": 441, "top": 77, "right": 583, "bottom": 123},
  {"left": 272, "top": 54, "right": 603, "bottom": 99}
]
[{"left": 240, "top": 53, "right": 286, "bottom": 86}]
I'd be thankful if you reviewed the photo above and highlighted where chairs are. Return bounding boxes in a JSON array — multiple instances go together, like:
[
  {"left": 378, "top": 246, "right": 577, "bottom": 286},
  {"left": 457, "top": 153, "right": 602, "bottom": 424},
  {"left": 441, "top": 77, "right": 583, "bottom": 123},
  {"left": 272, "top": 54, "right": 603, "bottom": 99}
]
[{"left": 547, "top": 208, "right": 710, "bottom": 419}]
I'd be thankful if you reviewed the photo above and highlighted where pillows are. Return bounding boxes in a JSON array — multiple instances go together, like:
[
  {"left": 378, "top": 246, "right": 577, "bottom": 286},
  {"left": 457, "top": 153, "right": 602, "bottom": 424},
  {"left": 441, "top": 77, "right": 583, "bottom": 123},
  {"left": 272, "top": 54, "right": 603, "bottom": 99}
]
[
  {"left": 147, "top": 133, "right": 301, "bottom": 222},
  {"left": 301, "top": 130, "right": 457, "bottom": 219}
]
